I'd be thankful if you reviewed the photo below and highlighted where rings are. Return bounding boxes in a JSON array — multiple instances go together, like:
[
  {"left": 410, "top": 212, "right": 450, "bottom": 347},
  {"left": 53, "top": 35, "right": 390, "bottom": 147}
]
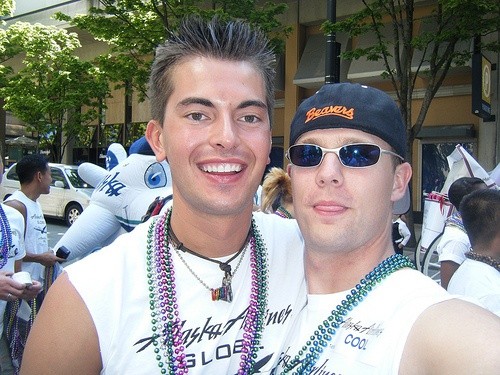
[{"left": 7, "top": 294, "right": 12, "bottom": 302}]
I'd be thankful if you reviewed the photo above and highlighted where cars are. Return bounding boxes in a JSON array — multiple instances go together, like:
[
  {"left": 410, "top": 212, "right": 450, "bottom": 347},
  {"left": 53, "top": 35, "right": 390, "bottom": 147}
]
[{"left": 1, "top": 162, "right": 95, "bottom": 226}]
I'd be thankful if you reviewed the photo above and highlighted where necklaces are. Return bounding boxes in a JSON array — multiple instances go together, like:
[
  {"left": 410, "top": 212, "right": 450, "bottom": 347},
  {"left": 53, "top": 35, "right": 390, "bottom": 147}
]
[
  {"left": 148, "top": 204, "right": 270, "bottom": 375},
  {"left": 278, "top": 254, "right": 418, "bottom": 375}
]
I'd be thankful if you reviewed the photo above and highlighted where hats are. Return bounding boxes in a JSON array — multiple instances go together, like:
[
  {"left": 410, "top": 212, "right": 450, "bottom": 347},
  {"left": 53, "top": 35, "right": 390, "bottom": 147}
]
[{"left": 289, "top": 82, "right": 407, "bottom": 160}]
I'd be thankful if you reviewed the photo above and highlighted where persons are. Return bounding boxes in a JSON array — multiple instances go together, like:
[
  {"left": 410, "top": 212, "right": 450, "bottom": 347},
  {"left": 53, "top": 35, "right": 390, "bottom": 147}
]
[
  {"left": 253, "top": 83, "right": 500, "bottom": 375},
  {"left": 0, "top": 146, "right": 45, "bottom": 375},
  {"left": 436, "top": 177, "right": 492, "bottom": 290},
  {"left": 256, "top": 166, "right": 297, "bottom": 220},
  {"left": 18, "top": 8, "right": 310, "bottom": 375},
  {"left": 390, "top": 214, "right": 411, "bottom": 256},
  {"left": 446, "top": 188, "right": 500, "bottom": 318},
  {"left": 2, "top": 152, "right": 69, "bottom": 357}
]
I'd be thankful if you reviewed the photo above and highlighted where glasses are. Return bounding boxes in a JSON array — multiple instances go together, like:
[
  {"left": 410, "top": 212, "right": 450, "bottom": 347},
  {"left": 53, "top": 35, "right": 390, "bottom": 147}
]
[{"left": 284, "top": 143, "right": 407, "bottom": 169}]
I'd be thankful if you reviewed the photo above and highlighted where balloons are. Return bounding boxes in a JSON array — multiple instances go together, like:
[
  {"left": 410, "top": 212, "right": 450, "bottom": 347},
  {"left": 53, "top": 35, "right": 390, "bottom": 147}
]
[{"left": 51, "top": 135, "right": 263, "bottom": 266}]
